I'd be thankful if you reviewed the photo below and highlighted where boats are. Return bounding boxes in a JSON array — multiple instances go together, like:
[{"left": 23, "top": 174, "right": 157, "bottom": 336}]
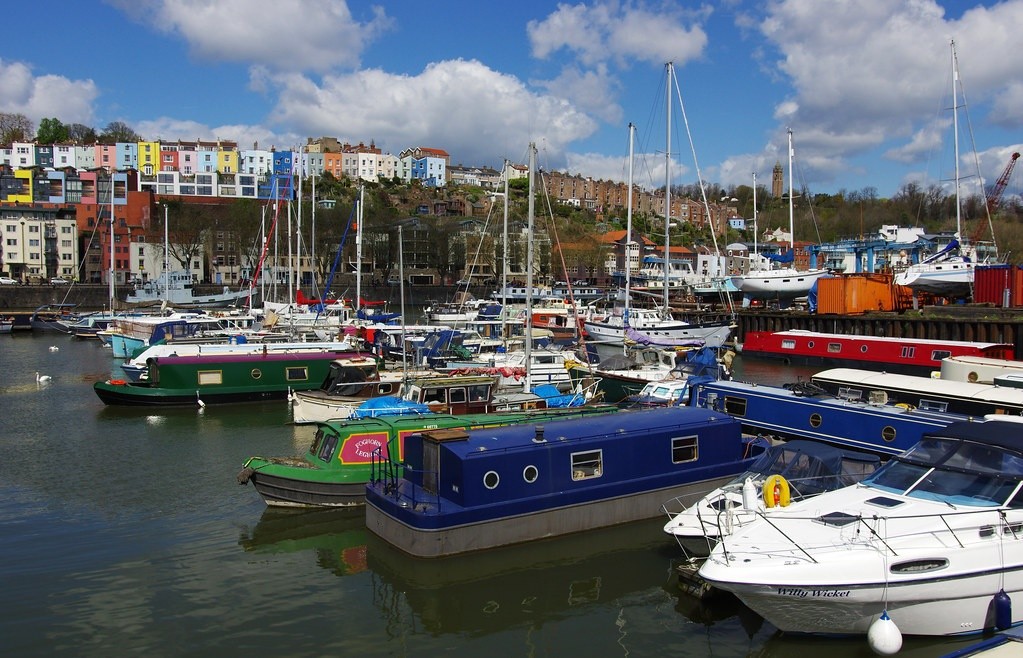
[
  {"left": 654, "top": 437, "right": 882, "bottom": 561},
  {"left": 695, "top": 414, "right": 1023, "bottom": 639}
]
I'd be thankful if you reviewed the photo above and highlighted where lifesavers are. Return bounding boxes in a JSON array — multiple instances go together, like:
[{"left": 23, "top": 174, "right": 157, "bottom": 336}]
[
  {"left": 762, "top": 474, "right": 791, "bottom": 508},
  {"left": 110, "top": 379, "right": 127, "bottom": 386}
]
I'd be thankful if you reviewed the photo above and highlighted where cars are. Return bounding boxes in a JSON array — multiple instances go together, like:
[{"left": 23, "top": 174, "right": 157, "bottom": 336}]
[
  {"left": 26, "top": 38, "right": 1023, "bottom": 658},
  {"left": 0, "top": 277, "right": 20, "bottom": 286},
  {"left": 455, "top": 279, "right": 472, "bottom": 287},
  {"left": 387, "top": 278, "right": 400, "bottom": 287}
]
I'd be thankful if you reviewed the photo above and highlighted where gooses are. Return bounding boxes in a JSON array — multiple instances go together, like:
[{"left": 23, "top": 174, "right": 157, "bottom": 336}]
[{"left": 35, "top": 372, "right": 51, "bottom": 381}]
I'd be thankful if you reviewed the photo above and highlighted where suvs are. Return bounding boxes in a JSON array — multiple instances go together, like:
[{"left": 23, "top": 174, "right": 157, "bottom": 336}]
[{"left": 50, "top": 277, "right": 70, "bottom": 286}]
